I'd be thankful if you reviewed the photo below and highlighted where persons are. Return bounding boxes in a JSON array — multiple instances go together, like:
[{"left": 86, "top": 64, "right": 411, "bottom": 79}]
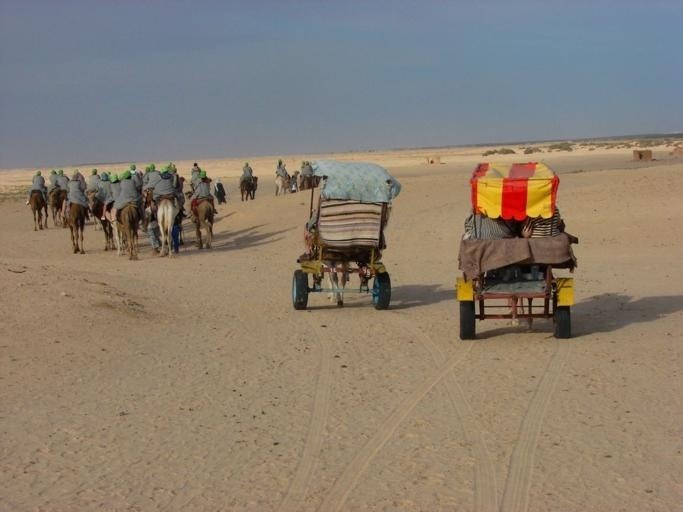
[
  {"left": 275, "top": 159, "right": 290, "bottom": 180},
  {"left": 289, "top": 175, "right": 297, "bottom": 193},
  {"left": 464, "top": 213, "right": 522, "bottom": 282},
  {"left": 214, "top": 178, "right": 227, "bottom": 204},
  {"left": 237, "top": 162, "right": 253, "bottom": 188},
  {"left": 520, "top": 204, "right": 561, "bottom": 281},
  {"left": 25, "top": 161, "right": 219, "bottom": 254},
  {"left": 298, "top": 161, "right": 318, "bottom": 188}
]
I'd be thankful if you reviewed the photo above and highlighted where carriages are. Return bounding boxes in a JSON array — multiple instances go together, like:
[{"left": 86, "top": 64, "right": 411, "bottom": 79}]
[
  {"left": 456, "top": 160, "right": 576, "bottom": 340},
  {"left": 292, "top": 159, "right": 401, "bottom": 311}
]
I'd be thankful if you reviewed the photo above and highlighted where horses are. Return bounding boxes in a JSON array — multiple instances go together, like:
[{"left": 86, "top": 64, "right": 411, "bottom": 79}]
[
  {"left": 27, "top": 178, "right": 215, "bottom": 258},
  {"left": 273, "top": 170, "right": 313, "bottom": 196},
  {"left": 238, "top": 176, "right": 258, "bottom": 202}
]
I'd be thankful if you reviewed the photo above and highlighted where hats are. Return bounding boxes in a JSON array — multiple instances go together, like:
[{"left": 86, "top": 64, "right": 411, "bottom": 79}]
[
  {"left": 59, "top": 170, "right": 62, "bottom": 174},
  {"left": 199, "top": 171, "right": 205, "bottom": 178},
  {"left": 91, "top": 163, "right": 176, "bottom": 183},
  {"left": 51, "top": 170, "right": 56, "bottom": 174},
  {"left": 36, "top": 171, "right": 41, "bottom": 175}
]
